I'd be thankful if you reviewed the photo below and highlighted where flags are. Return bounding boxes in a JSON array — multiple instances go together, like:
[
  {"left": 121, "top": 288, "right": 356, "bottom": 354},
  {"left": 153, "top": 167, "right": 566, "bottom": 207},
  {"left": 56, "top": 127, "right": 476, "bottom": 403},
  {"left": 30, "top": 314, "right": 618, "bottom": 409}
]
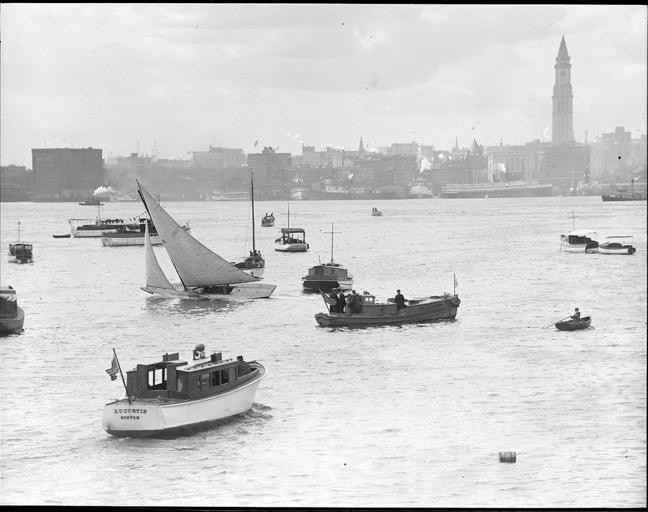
[
  {"left": 318, "top": 288, "right": 337, "bottom": 307},
  {"left": 454, "top": 274, "right": 458, "bottom": 289},
  {"left": 104, "top": 349, "right": 121, "bottom": 382}
]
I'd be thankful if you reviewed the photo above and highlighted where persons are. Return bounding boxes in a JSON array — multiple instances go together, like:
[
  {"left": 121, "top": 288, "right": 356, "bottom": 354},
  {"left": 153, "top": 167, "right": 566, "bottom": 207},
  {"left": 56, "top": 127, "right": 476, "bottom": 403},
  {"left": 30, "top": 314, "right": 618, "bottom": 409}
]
[
  {"left": 330, "top": 286, "right": 342, "bottom": 313},
  {"left": 570, "top": 307, "right": 581, "bottom": 323},
  {"left": 339, "top": 289, "right": 348, "bottom": 313},
  {"left": 394, "top": 289, "right": 405, "bottom": 311},
  {"left": 237, "top": 356, "right": 251, "bottom": 376}
]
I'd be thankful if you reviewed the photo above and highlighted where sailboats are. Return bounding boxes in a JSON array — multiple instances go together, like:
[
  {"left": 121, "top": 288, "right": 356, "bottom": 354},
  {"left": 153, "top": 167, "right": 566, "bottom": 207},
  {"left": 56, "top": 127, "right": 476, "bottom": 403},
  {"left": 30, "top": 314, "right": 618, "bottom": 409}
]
[{"left": 135, "top": 169, "right": 276, "bottom": 300}]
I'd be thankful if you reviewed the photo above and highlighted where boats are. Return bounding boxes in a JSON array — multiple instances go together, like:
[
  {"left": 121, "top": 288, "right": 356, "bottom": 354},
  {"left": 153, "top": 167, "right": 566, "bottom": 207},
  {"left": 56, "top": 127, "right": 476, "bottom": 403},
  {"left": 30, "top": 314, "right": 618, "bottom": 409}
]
[
  {"left": 370, "top": 207, "right": 383, "bottom": 217},
  {"left": 546, "top": 315, "right": 595, "bottom": 331},
  {"left": 559, "top": 233, "right": 636, "bottom": 255},
  {"left": 101, "top": 341, "right": 268, "bottom": 440},
  {"left": 274, "top": 200, "right": 311, "bottom": 252},
  {"left": 8, "top": 221, "right": 34, "bottom": 265},
  {"left": 313, "top": 274, "right": 462, "bottom": 327},
  {"left": 301, "top": 222, "right": 354, "bottom": 291},
  {"left": 0, "top": 283, "right": 25, "bottom": 336},
  {"left": 53, "top": 194, "right": 192, "bottom": 247},
  {"left": 601, "top": 189, "right": 647, "bottom": 202}
]
